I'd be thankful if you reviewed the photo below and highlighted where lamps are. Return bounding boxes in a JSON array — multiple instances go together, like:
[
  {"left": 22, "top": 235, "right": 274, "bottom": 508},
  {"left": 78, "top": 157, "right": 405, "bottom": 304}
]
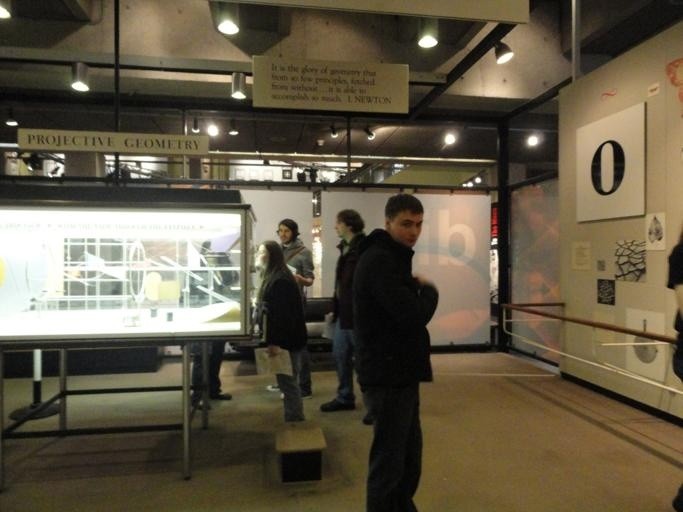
[
  {"left": 329, "top": 123, "right": 339, "bottom": 139},
  {"left": 190, "top": 113, "right": 241, "bottom": 139},
  {"left": 6, "top": 108, "right": 21, "bottom": 128},
  {"left": 474, "top": 172, "right": 488, "bottom": 184},
  {"left": 69, "top": 59, "right": 93, "bottom": 95},
  {"left": 214, "top": 2, "right": 242, "bottom": 36},
  {"left": 363, "top": 121, "right": 376, "bottom": 142},
  {"left": 227, "top": 71, "right": 248, "bottom": 102},
  {"left": 489, "top": 38, "right": 515, "bottom": 67},
  {"left": 10, "top": 149, "right": 61, "bottom": 178},
  {"left": 1, "top": 1, "right": 16, "bottom": 23},
  {"left": 416, "top": 14, "right": 445, "bottom": 48}
]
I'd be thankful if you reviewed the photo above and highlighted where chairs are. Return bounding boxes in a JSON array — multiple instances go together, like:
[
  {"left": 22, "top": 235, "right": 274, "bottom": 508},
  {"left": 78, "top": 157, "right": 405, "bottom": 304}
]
[{"left": 304, "top": 295, "right": 354, "bottom": 372}]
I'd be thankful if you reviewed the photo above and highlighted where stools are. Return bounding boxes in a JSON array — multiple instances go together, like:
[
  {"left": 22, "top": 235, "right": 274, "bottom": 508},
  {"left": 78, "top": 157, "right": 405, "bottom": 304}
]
[{"left": 273, "top": 418, "right": 328, "bottom": 487}]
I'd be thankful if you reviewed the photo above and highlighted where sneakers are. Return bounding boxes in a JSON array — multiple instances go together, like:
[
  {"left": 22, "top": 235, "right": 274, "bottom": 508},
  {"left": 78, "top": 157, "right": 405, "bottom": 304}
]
[
  {"left": 280, "top": 388, "right": 313, "bottom": 402},
  {"left": 267, "top": 383, "right": 281, "bottom": 393},
  {"left": 191, "top": 397, "right": 211, "bottom": 411},
  {"left": 320, "top": 396, "right": 356, "bottom": 413},
  {"left": 209, "top": 390, "right": 233, "bottom": 401}
]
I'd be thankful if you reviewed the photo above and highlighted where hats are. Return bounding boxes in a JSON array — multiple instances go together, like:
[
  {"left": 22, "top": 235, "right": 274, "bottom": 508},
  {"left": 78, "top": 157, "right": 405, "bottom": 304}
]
[{"left": 276, "top": 218, "right": 299, "bottom": 242}]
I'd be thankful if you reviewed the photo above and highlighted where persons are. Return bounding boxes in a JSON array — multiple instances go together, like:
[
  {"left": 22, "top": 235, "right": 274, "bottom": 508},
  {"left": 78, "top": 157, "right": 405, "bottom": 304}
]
[
  {"left": 266, "top": 219, "right": 315, "bottom": 399},
  {"left": 191, "top": 241, "right": 232, "bottom": 410},
  {"left": 353, "top": 194, "right": 441, "bottom": 511},
  {"left": 666, "top": 228, "right": 683, "bottom": 511},
  {"left": 319, "top": 208, "right": 376, "bottom": 426},
  {"left": 254, "top": 240, "right": 308, "bottom": 420}
]
